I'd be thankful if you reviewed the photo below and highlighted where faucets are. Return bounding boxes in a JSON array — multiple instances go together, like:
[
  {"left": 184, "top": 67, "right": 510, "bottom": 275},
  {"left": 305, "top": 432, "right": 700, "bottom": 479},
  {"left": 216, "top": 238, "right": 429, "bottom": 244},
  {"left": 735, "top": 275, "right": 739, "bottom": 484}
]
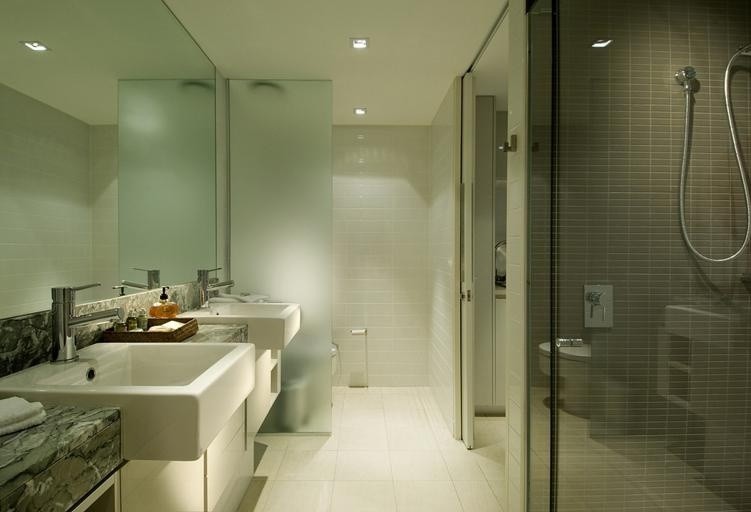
[
  {"left": 51, "top": 283, "right": 124, "bottom": 365},
  {"left": 197, "top": 267, "right": 234, "bottom": 308},
  {"left": 122, "top": 268, "right": 160, "bottom": 292}
]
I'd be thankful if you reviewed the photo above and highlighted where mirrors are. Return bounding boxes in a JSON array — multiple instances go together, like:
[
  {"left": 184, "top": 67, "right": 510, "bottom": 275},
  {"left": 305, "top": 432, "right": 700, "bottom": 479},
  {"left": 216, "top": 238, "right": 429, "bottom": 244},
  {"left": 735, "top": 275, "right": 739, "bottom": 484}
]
[{"left": 2, "top": 2, "right": 220, "bottom": 322}]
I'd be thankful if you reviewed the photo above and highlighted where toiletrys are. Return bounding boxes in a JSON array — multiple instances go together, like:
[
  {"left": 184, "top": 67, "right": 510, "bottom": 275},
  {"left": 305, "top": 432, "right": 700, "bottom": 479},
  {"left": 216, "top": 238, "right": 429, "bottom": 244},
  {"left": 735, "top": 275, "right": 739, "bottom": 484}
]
[{"left": 114, "top": 308, "right": 149, "bottom": 332}]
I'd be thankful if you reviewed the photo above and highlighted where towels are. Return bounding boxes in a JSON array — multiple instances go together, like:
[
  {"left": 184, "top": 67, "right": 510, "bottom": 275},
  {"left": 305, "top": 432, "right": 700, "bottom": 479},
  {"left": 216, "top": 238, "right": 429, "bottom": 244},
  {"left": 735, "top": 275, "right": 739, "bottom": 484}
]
[{"left": 1, "top": 396, "right": 47, "bottom": 435}]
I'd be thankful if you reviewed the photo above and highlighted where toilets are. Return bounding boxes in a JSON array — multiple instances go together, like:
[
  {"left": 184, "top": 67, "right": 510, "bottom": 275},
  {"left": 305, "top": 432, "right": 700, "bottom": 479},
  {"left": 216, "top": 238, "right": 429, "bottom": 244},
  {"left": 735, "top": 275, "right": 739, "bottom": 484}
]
[{"left": 538, "top": 341, "right": 592, "bottom": 418}]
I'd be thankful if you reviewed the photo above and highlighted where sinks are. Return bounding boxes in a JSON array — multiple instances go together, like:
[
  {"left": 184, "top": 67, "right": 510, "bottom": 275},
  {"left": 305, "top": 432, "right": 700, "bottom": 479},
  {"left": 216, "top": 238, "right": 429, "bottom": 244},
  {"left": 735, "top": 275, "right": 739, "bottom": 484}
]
[
  {"left": 0, "top": 342, "right": 256, "bottom": 461},
  {"left": 176, "top": 302, "right": 301, "bottom": 348},
  {"left": 664, "top": 303, "right": 741, "bottom": 349}
]
